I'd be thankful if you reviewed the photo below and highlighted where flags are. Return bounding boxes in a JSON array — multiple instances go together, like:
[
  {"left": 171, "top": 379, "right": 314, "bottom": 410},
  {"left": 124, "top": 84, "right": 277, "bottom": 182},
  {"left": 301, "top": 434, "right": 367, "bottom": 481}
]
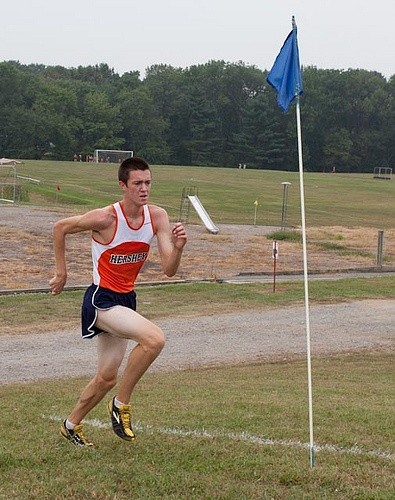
[{"left": 266, "top": 23, "right": 303, "bottom": 112}]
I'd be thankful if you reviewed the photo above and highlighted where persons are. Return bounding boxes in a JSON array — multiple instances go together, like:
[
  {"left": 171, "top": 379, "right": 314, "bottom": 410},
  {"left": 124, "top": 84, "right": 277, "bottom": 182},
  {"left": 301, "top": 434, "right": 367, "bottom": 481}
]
[
  {"left": 73, "top": 154, "right": 110, "bottom": 163},
  {"left": 49, "top": 157, "right": 188, "bottom": 447}
]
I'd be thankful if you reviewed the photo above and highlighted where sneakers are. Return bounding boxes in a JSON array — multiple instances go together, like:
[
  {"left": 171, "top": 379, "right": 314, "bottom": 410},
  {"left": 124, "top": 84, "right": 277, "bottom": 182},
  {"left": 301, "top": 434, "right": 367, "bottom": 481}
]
[
  {"left": 59, "top": 418, "right": 93, "bottom": 447},
  {"left": 108, "top": 395, "right": 135, "bottom": 442}
]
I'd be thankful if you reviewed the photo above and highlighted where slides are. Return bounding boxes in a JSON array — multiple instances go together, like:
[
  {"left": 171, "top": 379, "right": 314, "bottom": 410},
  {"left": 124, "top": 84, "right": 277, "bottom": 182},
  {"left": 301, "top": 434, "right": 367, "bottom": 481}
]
[{"left": 187, "top": 194, "right": 219, "bottom": 233}]
